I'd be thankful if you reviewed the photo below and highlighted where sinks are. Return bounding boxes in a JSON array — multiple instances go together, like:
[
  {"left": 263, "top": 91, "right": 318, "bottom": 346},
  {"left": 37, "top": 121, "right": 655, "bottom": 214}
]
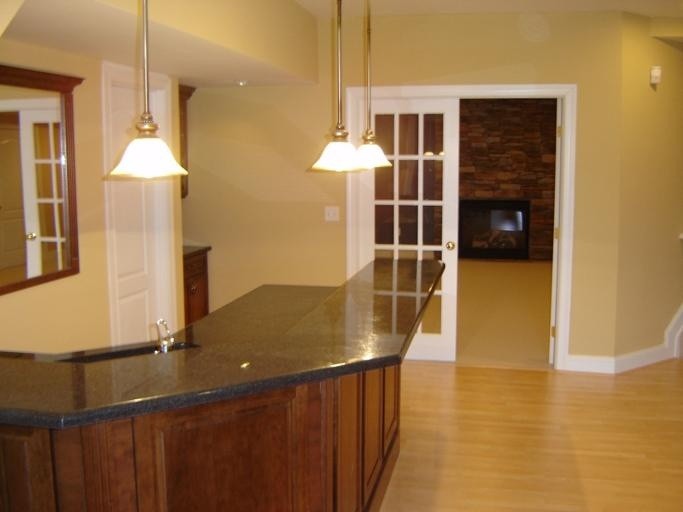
[{"left": 58, "top": 342, "right": 196, "bottom": 363}]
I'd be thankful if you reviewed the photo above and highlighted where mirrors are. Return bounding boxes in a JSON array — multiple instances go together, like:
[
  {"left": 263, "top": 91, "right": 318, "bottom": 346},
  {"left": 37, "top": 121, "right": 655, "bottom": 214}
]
[{"left": 0, "top": 64, "right": 80, "bottom": 296}]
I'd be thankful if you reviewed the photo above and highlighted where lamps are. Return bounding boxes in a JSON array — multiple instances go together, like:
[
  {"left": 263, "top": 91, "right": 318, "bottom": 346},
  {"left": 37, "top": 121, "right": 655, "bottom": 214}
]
[
  {"left": 356, "top": 0, "right": 393, "bottom": 170},
  {"left": 108, "top": 0, "right": 190, "bottom": 180},
  {"left": 309, "top": 0, "right": 367, "bottom": 174}
]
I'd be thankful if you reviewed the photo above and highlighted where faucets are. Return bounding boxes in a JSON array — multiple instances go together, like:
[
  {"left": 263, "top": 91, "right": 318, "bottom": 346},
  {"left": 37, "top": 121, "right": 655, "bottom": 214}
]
[{"left": 156, "top": 318, "right": 168, "bottom": 344}]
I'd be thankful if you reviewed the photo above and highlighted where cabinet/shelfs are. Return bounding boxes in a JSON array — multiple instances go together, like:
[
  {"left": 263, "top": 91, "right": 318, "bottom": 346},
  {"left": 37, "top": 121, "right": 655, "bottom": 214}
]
[
  {"left": 184, "top": 246, "right": 210, "bottom": 325},
  {"left": 178, "top": 86, "right": 193, "bottom": 200}
]
